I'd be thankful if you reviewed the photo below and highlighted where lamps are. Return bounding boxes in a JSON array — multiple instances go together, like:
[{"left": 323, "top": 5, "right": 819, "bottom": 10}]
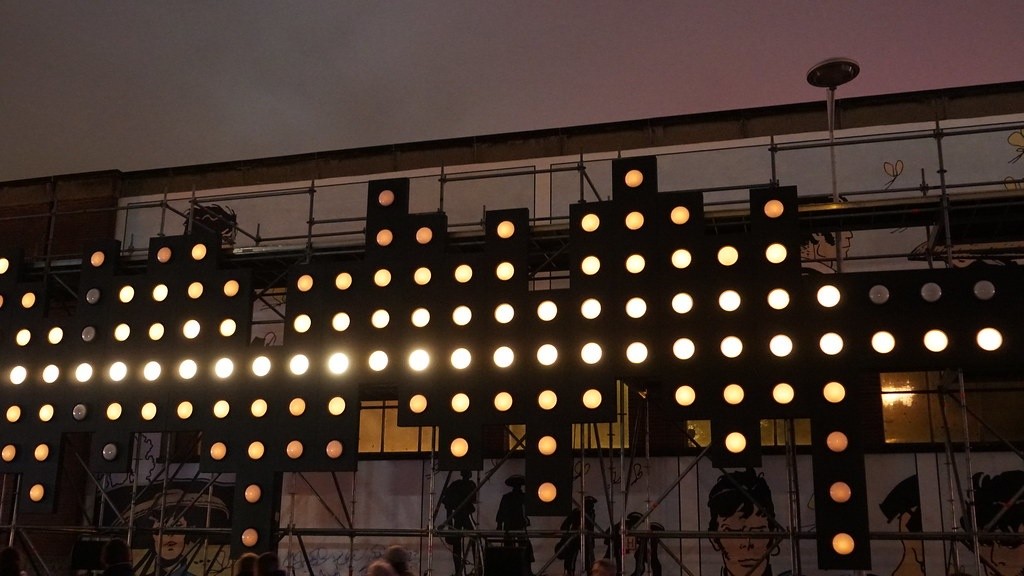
[{"left": 3, "top": 155, "right": 1024, "bottom": 570}]
